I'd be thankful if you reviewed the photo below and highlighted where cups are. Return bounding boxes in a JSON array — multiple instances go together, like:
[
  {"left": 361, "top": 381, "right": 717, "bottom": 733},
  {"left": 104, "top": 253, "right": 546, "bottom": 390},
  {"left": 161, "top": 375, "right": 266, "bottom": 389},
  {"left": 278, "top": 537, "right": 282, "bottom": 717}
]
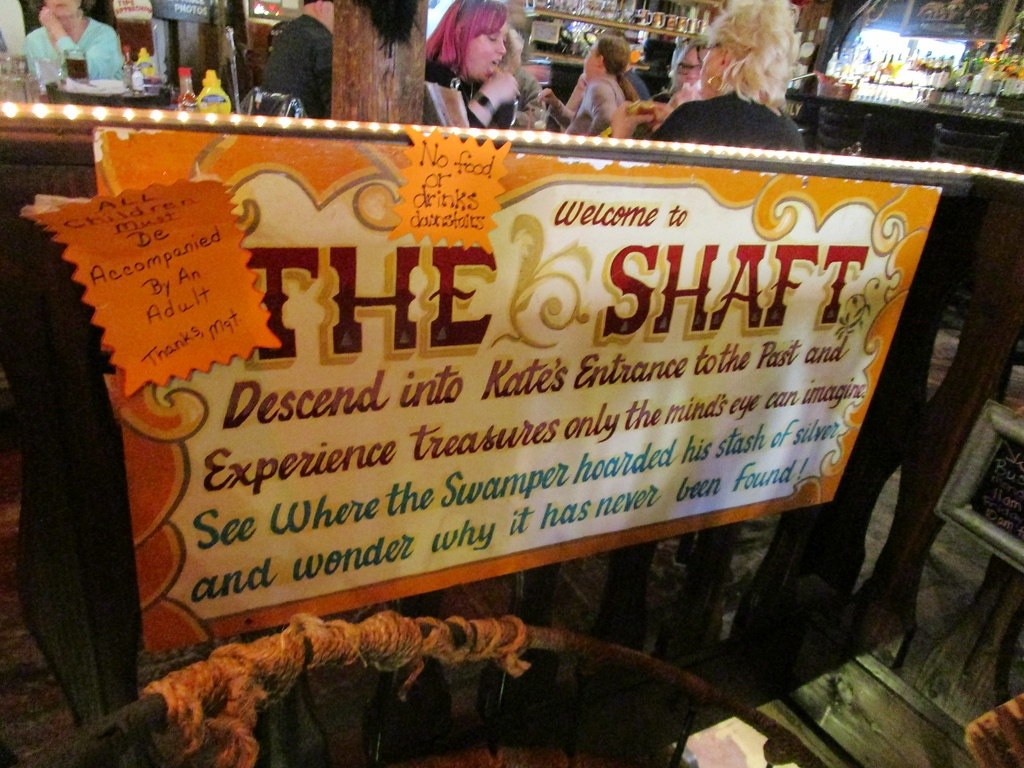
[
  {"left": 6, "top": 54, "right": 30, "bottom": 76},
  {"left": 0, "top": 75, "right": 33, "bottom": 103},
  {"left": 528, "top": 103, "right": 551, "bottom": 131},
  {"left": 35, "top": 60, "right": 62, "bottom": 103},
  {"left": 537, "top": 0, "right": 702, "bottom": 34},
  {"left": 65, "top": 49, "right": 89, "bottom": 84}
]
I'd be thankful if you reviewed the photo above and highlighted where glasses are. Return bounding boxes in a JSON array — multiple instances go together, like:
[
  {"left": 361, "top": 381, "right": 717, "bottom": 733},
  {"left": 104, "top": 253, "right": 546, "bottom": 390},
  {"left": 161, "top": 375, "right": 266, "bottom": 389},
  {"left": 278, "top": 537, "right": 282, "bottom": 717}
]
[{"left": 677, "top": 62, "right": 702, "bottom": 74}]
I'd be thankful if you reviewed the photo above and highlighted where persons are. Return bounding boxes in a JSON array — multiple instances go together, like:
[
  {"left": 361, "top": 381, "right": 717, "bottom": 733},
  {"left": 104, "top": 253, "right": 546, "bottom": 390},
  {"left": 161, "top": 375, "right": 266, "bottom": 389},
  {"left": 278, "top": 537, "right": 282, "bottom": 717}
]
[
  {"left": 259, "top": 1, "right": 809, "bottom": 152},
  {"left": 21, "top": 0, "right": 124, "bottom": 83}
]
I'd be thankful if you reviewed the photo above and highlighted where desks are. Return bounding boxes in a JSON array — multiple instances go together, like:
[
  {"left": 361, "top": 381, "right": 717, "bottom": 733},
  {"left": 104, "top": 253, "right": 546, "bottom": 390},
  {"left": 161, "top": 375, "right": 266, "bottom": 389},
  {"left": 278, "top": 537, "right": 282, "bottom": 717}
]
[{"left": 46, "top": 75, "right": 175, "bottom": 111}]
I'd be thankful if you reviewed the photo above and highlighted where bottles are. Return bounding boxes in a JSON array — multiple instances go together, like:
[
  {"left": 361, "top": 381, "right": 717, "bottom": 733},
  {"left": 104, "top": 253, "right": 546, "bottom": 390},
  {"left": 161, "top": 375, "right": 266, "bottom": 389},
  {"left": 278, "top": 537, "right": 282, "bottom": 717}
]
[
  {"left": 825, "top": 45, "right": 1024, "bottom": 119},
  {"left": 122, "top": 45, "right": 155, "bottom": 92},
  {"left": 177, "top": 67, "right": 197, "bottom": 112},
  {"left": 197, "top": 70, "right": 231, "bottom": 113}
]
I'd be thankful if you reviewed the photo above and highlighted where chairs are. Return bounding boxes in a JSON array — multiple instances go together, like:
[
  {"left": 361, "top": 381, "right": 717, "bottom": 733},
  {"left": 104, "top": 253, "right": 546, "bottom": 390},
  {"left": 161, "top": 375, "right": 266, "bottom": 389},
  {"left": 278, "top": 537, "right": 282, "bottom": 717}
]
[
  {"left": 815, "top": 107, "right": 873, "bottom": 155},
  {"left": 928, "top": 122, "right": 1010, "bottom": 170},
  {"left": 238, "top": 86, "right": 306, "bottom": 119}
]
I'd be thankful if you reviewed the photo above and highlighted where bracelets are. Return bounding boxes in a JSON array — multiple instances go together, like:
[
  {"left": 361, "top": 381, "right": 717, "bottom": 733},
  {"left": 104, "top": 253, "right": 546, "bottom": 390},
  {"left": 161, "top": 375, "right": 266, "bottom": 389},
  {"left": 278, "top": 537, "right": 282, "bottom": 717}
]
[{"left": 473, "top": 91, "right": 497, "bottom": 117}]
